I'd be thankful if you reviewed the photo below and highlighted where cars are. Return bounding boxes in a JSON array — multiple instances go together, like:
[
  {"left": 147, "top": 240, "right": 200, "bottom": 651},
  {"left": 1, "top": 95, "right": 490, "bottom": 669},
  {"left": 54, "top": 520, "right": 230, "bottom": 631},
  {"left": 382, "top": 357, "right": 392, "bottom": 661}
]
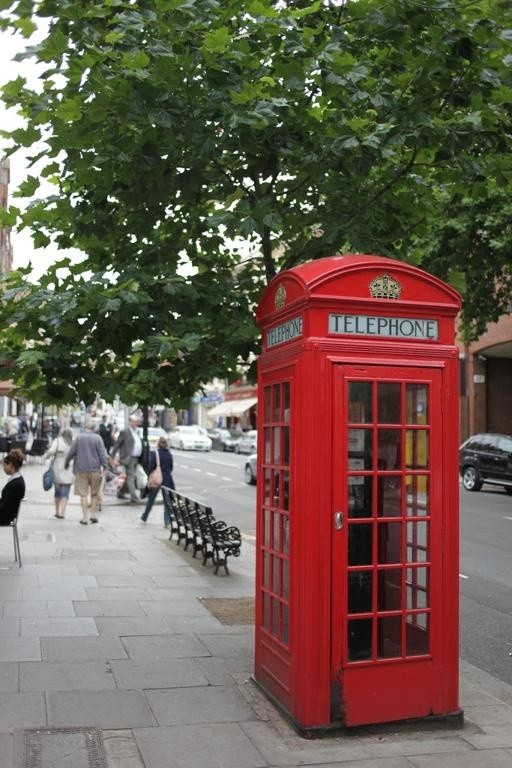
[{"left": 132, "top": 422, "right": 277, "bottom": 488}]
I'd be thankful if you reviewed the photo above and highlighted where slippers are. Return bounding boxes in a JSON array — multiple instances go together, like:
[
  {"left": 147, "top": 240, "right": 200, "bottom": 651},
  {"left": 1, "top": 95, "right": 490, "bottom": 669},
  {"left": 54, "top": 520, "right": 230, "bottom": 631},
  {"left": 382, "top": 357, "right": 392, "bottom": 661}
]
[
  {"left": 90, "top": 518, "right": 98, "bottom": 522},
  {"left": 54, "top": 512, "right": 66, "bottom": 520},
  {"left": 79, "top": 519, "right": 87, "bottom": 524}
]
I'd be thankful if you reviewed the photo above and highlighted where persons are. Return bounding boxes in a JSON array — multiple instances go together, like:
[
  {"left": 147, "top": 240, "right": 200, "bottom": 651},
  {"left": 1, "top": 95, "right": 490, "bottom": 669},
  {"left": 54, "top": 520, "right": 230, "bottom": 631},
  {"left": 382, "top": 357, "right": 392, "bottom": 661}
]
[{"left": 0, "top": 407, "right": 177, "bottom": 527}]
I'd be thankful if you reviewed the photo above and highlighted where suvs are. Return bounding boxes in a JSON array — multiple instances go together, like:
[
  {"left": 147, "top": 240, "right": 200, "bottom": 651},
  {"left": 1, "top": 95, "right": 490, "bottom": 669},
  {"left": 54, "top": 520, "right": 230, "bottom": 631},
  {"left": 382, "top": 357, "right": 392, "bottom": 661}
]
[{"left": 459, "top": 430, "right": 511, "bottom": 494}]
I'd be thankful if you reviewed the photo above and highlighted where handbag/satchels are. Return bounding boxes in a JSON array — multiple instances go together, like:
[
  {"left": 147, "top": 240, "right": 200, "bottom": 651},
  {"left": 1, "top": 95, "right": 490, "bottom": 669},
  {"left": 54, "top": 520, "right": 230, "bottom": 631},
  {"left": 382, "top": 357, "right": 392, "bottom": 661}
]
[
  {"left": 42, "top": 468, "right": 54, "bottom": 491},
  {"left": 135, "top": 462, "right": 148, "bottom": 490},
  {"left": 146, "top": 465, "right": 163, "bottom": 490}
]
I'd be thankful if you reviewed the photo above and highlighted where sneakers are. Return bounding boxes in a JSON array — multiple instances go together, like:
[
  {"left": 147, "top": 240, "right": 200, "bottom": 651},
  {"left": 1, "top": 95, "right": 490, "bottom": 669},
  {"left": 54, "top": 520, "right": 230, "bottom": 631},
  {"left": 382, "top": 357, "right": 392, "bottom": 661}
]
[
  {"left": 117, "top": 495, "right": 130, "bottom": 500},
  {"left": 139, "top": 516, "right": 147, "bottom": 523},
  {"left": 165, "top": 523, "right": 172, "bottom": 529},
  {"left": 131, "top": 499, "right": 144, "bottom": 504}
]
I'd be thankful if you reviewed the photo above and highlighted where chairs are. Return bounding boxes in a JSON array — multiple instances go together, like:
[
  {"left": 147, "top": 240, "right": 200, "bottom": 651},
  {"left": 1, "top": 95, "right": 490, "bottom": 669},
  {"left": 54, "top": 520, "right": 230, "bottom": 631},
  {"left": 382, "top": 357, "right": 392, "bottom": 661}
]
[
  {"left": 0, "top": 434, "right": 50, "bottom": 469},
  {"left": 0, "top": 497, "right": 29, "bottom": 567},
  {"left": 161, "top": 485, "right": 242, "bottom": 575}
]
